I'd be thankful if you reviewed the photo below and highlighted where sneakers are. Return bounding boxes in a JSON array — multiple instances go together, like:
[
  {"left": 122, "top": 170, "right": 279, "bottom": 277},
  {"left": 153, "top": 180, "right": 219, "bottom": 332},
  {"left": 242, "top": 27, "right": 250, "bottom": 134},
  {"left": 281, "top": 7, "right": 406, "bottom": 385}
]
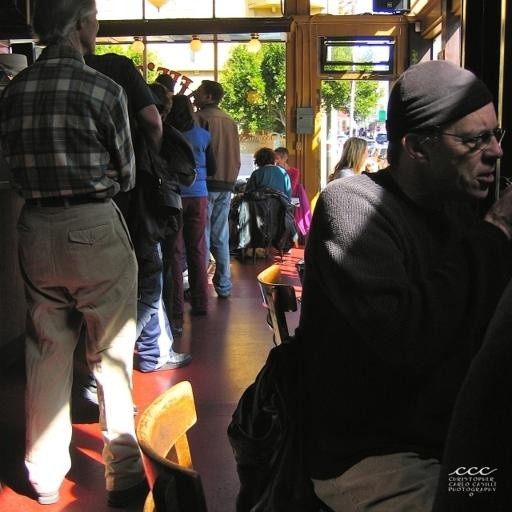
[
  {"left": 79, "top": 384, "right": 138, "bottom": 416},
  {"left": 26, "top": 483, "right": 59, "bottom": 504},
  {"left": 184, "top": 288, "right": 191, "bottom": 299},
  {"left": 245, "top": 248, "right": 265, "bottom": 258},
  {"left": 106, "top": 478, "right": 148, "bottom": 511},
  {"left": 160, "top": 349, "right": 191, "bottom": 369}
]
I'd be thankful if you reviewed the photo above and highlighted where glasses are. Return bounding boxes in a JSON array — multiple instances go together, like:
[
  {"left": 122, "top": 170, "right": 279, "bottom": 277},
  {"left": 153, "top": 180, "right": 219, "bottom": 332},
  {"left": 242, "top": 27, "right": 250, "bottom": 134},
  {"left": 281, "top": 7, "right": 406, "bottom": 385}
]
[{"left": 447, "top": 129, "right": 506, "bottom": 150}]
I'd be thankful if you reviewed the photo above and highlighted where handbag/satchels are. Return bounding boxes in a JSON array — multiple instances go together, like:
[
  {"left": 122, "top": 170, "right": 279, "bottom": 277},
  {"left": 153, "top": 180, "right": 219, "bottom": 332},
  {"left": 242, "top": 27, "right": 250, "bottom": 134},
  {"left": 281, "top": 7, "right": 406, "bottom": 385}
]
[
  {"left": 127, "top": 169, "right": 182, "bottom": 248},
  {"left": 229, "top": 333, "right": 308, "bottom": 511}
]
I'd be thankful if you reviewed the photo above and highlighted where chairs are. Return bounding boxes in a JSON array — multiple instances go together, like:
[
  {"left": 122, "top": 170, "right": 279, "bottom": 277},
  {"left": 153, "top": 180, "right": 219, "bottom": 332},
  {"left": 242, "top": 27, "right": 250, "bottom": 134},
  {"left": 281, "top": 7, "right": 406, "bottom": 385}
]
[
  {"left": 235, "top": 184, "right": 305, "bottom": 265},
  {"left": 257, "top": 265, "right": 298, "bottom": 347},
  {"left": 138, "top": 381, "right": 208, "bottom": 512}
]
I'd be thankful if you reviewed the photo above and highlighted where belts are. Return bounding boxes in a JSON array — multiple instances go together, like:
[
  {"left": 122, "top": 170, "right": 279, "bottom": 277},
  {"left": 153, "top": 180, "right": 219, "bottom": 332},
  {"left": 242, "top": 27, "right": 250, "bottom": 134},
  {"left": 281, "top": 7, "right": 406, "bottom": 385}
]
[{"left": 26, "top": 196, "right": 103, "bottom": 207}]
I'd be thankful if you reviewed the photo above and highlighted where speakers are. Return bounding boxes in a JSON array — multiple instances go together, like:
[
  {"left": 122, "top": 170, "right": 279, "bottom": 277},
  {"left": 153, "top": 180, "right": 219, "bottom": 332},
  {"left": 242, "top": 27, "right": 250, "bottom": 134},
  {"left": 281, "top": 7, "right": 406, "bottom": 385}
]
[{"left": 373, "top": 0, "right": 410, "bottom": 12}]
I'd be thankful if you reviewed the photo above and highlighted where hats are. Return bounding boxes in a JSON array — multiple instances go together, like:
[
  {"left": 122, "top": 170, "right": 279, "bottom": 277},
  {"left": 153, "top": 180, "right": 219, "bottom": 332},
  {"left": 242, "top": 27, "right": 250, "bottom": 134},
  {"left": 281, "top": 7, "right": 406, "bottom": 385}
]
[{"left": 386, "top": 61, "right": 493, "bottom": 141}]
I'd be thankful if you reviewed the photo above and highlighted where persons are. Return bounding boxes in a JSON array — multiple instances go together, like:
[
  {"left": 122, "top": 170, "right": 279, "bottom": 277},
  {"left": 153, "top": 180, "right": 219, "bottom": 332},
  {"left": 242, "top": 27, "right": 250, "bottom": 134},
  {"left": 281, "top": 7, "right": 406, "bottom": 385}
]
[{"left": 0, "top": 1, "right": 511, "bottom": 511}]
